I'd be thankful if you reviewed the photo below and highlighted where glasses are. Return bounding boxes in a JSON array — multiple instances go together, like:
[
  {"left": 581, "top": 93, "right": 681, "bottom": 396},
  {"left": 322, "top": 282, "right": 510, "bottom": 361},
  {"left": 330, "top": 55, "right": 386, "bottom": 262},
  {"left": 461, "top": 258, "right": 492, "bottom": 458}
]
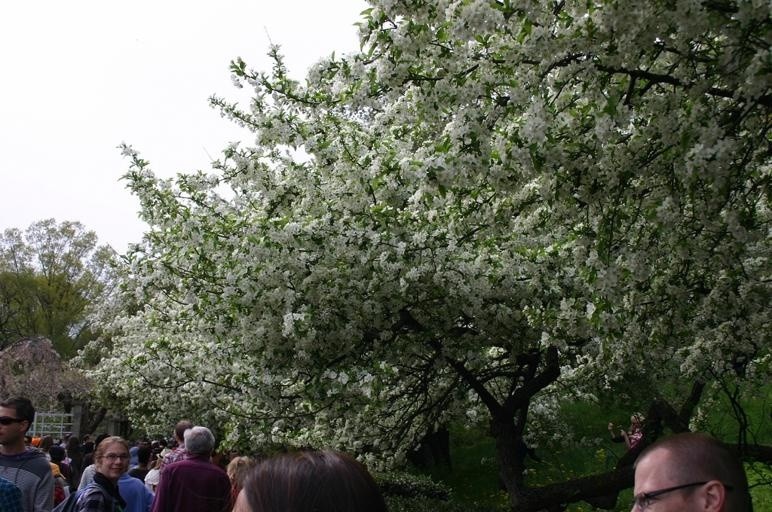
[
  {"left": 101, "top": 452, "right": 126, "bottom": 460},
  {"left": 0, "top": 416, "right": 19, "bottom": 426},
  {"left": 636, "top": 481, "right": 706, "bottom": 509}
]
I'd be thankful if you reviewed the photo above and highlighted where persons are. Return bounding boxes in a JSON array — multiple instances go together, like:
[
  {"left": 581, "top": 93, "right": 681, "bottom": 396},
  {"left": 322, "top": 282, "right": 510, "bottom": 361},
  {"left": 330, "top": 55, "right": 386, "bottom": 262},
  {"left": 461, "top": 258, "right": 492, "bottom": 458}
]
[
  {"left": 608, "top": 413, "right": 646, "bottom": 452},
  {"left": 25, "top": 419, "right": 388, "bottom": 511},
  {"left": 630, "top": 432, "right": 753, "bottom": 512},
  {"left": 2, "top": 398, "right": 54, "bottom": 511}
]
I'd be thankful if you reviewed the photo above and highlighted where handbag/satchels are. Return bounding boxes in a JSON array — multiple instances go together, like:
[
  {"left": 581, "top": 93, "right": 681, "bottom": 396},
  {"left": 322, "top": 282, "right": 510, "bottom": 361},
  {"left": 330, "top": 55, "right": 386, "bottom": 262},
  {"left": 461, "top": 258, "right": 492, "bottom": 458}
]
[{"left": 52, "top": 485, "right": 110, "bottom": 512}]
